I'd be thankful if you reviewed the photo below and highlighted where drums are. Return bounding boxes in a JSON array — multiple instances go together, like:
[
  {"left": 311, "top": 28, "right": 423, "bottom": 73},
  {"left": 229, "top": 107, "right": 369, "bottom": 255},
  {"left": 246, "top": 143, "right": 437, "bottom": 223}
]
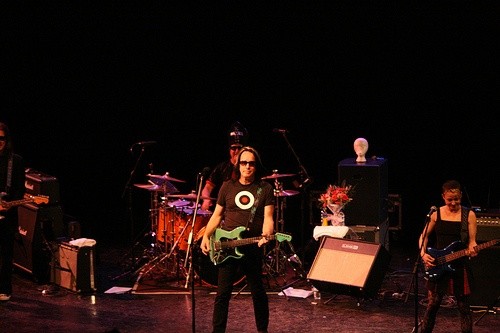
[
  {"left": 174, "top": 208, "right": 213, "bottom": 254},
  {"left": 155, "top": 199, "right": 201, "bottom": 246}
]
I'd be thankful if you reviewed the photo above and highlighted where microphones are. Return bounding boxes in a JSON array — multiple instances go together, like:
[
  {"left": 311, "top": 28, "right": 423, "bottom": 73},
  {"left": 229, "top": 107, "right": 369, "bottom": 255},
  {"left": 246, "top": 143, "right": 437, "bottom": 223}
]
[
  {"left": 137, "top": 141, "right": 157, "bottom": 145},
  {"left": 371, "top": 155, "right": 384, "bottom": 160},
  {"left": 56, "top": 266, "right": 71, "bottom": 272},
  {"left": 427, "top": 206, "right": 438, "bottom": 217},
  {"left": 272, "top": 128, "right": 289, "bottom": 134},
  {"left": 198, "top": 167, "right": 211, "bottom": 177}
]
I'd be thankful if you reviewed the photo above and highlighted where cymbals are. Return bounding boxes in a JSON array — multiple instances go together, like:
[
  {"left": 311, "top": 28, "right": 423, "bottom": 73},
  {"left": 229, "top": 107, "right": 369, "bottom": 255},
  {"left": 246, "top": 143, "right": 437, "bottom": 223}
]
[
  {"left": 273, "top": 189, "right": 300, "bottom": 196},
  {"left": 145, "top": 173, "right": 185, "bottom": 183},
  {"left": 132, "top": 183, "right": 180, "bottom": 194},
  {"left": 162, "top": 193, "right": 216, "bottom": 201},
  {"left": 262, "top": 172, "right": 297, "bottom": 179}
]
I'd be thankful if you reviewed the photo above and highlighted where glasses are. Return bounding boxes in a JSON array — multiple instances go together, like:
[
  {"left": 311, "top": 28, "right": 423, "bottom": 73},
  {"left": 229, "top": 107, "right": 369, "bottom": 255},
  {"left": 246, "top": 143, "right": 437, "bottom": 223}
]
[
  {"left": 239, "top": 161, "right": 257, "bottom": 167},
  {"left": 0, "top": 136, "right": 7, "bottom": 140},
  {"left": 230, "top": 146, "right": 243, "bottom": 150}
]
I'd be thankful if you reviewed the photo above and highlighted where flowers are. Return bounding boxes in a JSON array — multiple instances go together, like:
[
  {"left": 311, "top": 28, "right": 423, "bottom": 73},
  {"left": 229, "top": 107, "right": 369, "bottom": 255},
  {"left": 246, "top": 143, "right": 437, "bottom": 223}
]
[{"left": 318, "top": 184, "right": 353, "bottom": 214}]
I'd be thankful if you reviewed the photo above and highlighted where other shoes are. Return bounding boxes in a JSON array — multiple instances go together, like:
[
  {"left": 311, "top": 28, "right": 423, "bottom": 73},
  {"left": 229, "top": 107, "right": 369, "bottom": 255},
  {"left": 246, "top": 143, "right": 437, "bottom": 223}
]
[{"left": 0, "top": 293, "right": 12, "bottom": 301}]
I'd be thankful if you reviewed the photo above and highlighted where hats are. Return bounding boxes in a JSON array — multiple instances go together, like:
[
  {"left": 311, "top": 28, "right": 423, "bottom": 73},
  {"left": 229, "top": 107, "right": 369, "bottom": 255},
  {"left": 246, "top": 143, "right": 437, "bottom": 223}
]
[{"left": 227, "top": 127, "right": 247, "bottom": 151}]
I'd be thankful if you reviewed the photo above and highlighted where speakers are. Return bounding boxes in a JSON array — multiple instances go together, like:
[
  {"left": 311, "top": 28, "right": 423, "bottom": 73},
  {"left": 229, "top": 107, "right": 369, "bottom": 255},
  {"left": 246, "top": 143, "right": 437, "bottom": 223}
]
[
  {"left": 307, "top": 157, "right": 401, "bottom": 301},
  {"left": 466, "top": 246, "right": 500, "bottom": 308},
  {"left": 13, "top": 202, "right": 96, "bottom": 293}
]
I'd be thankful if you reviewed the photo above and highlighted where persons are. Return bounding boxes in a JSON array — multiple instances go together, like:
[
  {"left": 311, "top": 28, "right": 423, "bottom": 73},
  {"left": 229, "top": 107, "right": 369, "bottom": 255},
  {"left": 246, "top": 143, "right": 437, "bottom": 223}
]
[
  {"left": 201, "top": 132, "right": 243, "bottom": 209},
  {"left": 0, "top": 123, "right": 25, "bottom": 300},
  {"left": 419, "top": 182, "right": 478, "bottom": 333},
  {"left": 200, "top": 147, "right": 274, "bottom": 333}
]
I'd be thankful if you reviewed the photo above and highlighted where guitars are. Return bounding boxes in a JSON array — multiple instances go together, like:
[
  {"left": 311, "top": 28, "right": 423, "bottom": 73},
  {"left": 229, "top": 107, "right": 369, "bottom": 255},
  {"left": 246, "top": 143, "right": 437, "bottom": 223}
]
[
  {"left": 0, "top": 192, "right": 50, "bottom": 212},
  {"left": 205, "top": 225, "right": 293, "bottom": 267},
  {"left": 420, "top": 237, "right": 500, "bottom": 284}
]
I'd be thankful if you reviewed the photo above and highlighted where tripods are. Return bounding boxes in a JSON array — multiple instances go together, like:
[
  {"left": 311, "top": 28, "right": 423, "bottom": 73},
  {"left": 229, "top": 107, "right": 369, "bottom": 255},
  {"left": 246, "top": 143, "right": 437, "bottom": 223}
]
[
  {"left": 262, "top": 178, "right": 303, "bottom": 279},
  {"left": 133, "top": 179, "right": 192, "bottom": 286}
]
